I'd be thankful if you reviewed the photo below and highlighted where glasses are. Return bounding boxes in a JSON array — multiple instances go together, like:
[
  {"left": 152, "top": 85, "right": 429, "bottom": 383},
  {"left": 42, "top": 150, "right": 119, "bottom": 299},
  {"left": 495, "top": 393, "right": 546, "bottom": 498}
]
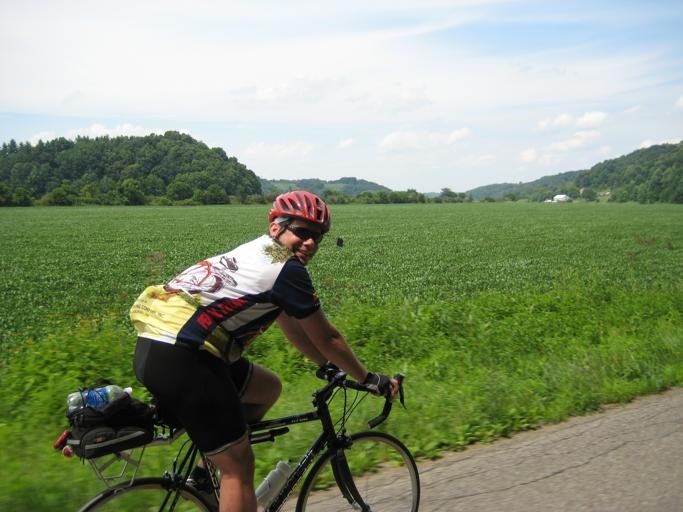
[{"left": 286, "top": 225, "right": 323, "bottom": 244}]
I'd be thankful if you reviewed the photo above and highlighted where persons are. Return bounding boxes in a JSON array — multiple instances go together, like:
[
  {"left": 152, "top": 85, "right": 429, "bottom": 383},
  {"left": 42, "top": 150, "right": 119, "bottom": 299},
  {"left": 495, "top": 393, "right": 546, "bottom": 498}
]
[{"left": 128, "top": 190, "right": 398, "bottom": 511}]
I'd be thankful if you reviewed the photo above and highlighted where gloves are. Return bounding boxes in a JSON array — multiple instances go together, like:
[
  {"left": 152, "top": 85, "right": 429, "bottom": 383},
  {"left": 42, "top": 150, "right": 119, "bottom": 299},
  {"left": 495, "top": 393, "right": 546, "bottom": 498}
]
[
  {"left": 358, "top": 372, "right": 395, "bottom": 398},
  {"left": 318, "top": 361, "right": 341, "bottom": 375}
]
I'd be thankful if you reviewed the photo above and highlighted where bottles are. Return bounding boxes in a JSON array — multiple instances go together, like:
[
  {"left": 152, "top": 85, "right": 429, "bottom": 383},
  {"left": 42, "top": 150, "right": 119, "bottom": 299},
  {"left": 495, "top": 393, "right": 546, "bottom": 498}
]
[
  {"left": 255, "top": 459, "right": 291, "bottom": 508},
  {"left": 67, "top": 385, "right": 134, "bottom": 414}
]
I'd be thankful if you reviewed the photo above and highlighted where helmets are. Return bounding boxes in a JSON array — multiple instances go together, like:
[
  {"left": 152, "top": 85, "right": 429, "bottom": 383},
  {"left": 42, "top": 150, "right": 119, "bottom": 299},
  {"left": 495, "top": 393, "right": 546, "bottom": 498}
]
[{"left": 268, "top": 190, "right": 332, "bottom": 234}]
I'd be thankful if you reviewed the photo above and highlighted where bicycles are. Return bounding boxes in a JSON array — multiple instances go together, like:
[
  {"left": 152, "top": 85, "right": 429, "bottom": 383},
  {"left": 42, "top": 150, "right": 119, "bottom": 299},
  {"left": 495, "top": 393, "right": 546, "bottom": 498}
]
[{"left": 53, "top": 361, "right": 421, "bottom": 512}]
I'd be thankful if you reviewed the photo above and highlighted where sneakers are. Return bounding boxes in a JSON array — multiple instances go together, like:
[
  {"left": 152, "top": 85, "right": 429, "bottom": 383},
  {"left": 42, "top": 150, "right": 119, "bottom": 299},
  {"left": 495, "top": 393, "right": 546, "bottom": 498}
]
[{"left": 178, "top": 475, "right": 219, "bottom": 512}]
